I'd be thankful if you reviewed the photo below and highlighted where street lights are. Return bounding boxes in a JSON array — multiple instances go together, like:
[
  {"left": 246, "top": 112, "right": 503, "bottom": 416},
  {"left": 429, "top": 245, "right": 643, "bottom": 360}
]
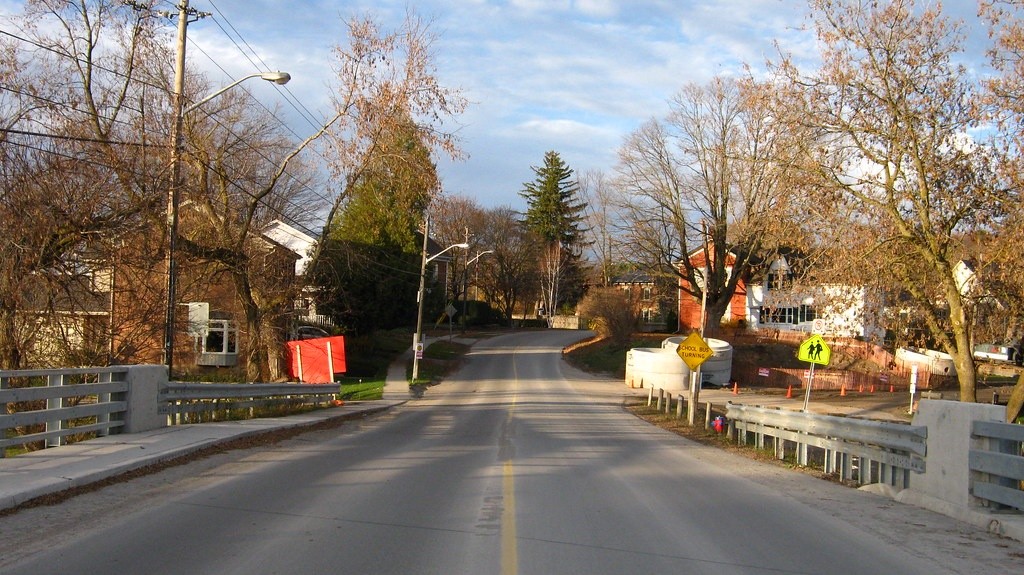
[
  {"left": 165, "top": 71, "right": 292, "bottom": 381},
  {"left": 410, "top": 244, "right": 472, "bottom": 387},
  {"left": 462, "top": 250, "right": 494, "bottom": 334}
]
